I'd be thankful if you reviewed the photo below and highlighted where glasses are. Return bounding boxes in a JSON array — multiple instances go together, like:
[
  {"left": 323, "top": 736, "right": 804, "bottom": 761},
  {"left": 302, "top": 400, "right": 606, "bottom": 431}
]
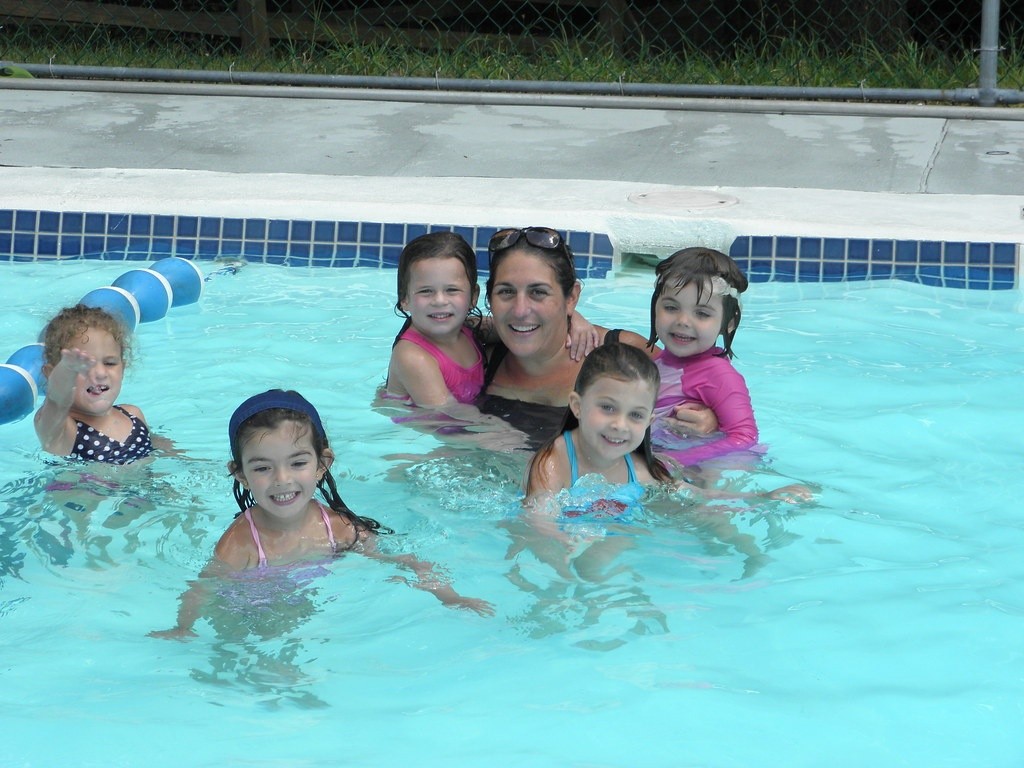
[
  {"left": 487, "top": 225, "right": 576, "bottom": 283},
  {"left": 653, "top": 267, "right": 742, "bottom": 301}
]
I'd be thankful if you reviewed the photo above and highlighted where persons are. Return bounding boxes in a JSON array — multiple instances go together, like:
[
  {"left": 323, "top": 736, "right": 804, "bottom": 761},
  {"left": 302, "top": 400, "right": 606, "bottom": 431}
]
[
  {"left": 34, "top": 303, "right": 178, "bottom": 535},
  {"left": 143, "top": 389, "right": 495, "bottom": 680},
  {"left": 372, "top": 227, "right": 812, "bottom": 583}
]
